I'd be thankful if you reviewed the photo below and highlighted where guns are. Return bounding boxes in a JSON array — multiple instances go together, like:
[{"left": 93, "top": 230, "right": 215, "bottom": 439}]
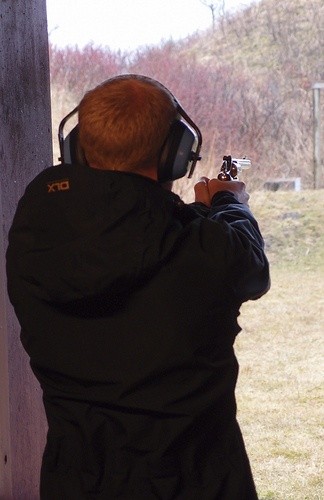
[{"left": 216, "top": 155, "right": 252, "bottom": 181}]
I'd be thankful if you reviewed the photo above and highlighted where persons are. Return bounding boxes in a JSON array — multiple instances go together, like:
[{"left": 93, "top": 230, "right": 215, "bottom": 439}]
[{"left": 7, "top": 72, "right": 271, "bottom": 499}]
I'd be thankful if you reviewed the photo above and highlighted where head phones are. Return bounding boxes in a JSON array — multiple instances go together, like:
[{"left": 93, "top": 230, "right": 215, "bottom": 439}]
[{"left": 58, "top": 75, "right": 203, "bottom": 183}]
